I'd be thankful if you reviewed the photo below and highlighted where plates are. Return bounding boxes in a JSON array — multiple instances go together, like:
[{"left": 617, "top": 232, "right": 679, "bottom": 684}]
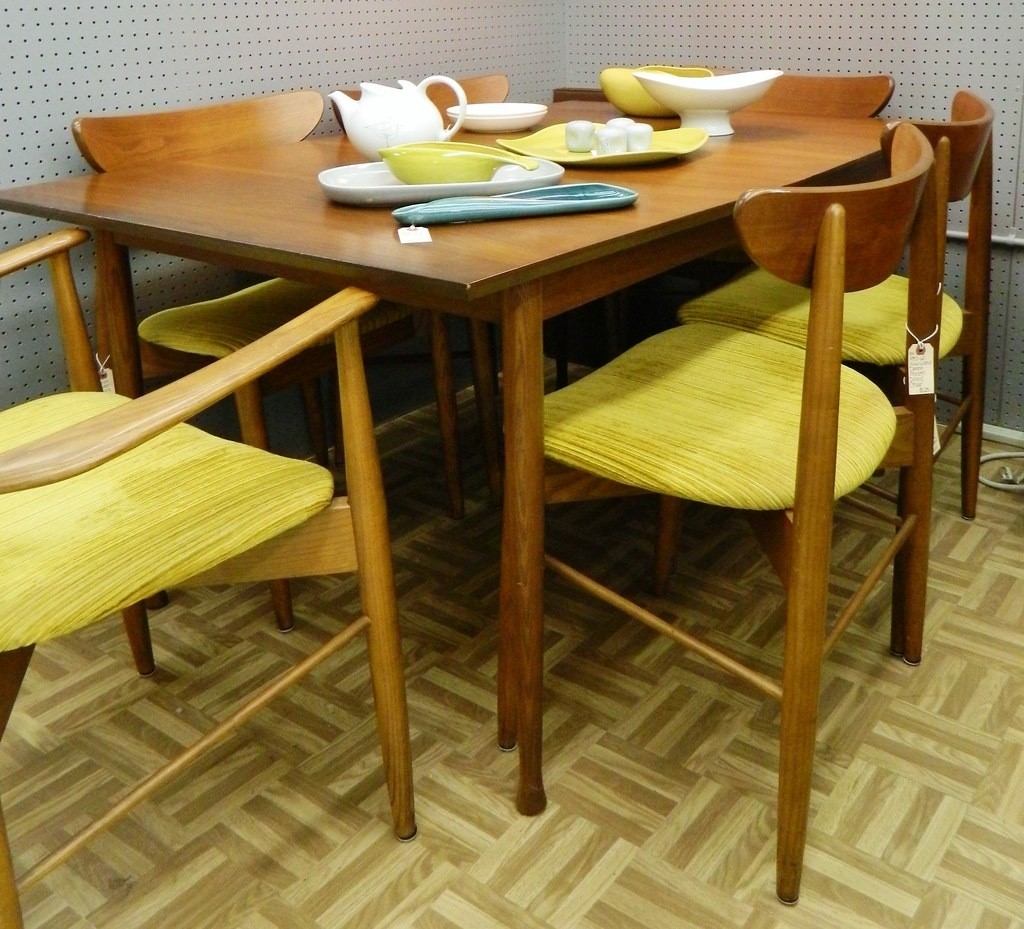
[
  {"left": 378, "top": 142, "right": 538, "bottom": 184},
  {"left": 446, "top": 103, "right": 547, "bottom": 134},
  {"left": 391, "top": 183, "right": 639, "bottom": 223},
  {"left": 496, "top": 121, "right": 708, "bottom": 164},
  {"left": 317, "top": 157, "right": 565, "bottom": 206}
]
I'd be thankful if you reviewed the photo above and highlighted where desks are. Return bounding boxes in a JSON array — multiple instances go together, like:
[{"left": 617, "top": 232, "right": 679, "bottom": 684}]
[{"left": 1, "top": 99, "right": 904, "bottom": 818}]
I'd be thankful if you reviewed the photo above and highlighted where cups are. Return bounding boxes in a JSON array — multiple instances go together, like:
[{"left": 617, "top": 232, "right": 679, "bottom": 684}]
[{"left": 565, "top": 119, "right": 653, "bottom": 156}]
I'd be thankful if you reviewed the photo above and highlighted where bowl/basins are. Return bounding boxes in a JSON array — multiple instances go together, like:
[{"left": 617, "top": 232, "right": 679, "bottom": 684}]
[
  {"left": 631, "top": 70, "right": 785, "bottom": 136},
  {"left": 600, "top": 65, "right": 714, "bottom": 117}
]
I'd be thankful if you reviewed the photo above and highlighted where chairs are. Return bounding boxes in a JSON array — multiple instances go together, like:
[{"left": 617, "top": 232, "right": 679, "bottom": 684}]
[
  {"left": 0, "top": 223, "right": 416, "bottom": 929},
  {"left": 657, "top": 91, "right": 997, "bottom": 653},
  {"left": 494, "top": 120, "right": 952, "bottom": 908},
  {"left": 70, "top": 73, "right": 895, "bottom": 635}
]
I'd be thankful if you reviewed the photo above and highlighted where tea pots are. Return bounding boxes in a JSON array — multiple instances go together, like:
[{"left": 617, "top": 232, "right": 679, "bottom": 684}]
[{"left": 328, "top": 75, "right": 467, "bottom": 161}]
